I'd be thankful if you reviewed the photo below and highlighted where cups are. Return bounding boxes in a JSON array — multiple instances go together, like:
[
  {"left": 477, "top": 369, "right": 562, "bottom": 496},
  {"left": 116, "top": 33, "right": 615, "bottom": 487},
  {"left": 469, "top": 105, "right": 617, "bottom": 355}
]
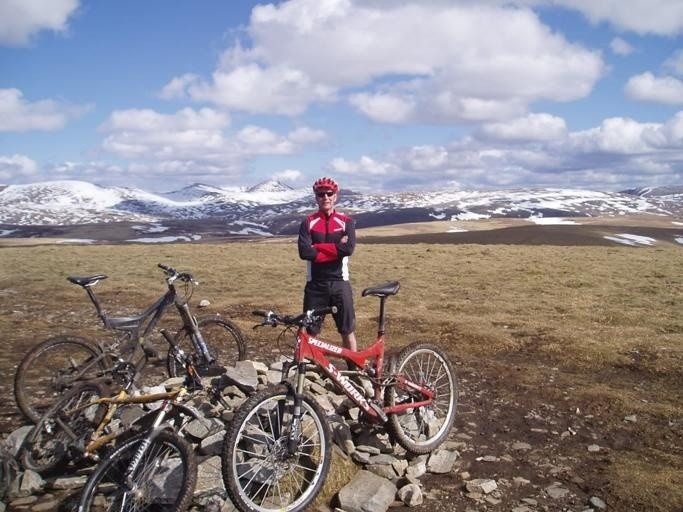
[{"left": 315, "top": 191, "right": 333, "bottom": 197}]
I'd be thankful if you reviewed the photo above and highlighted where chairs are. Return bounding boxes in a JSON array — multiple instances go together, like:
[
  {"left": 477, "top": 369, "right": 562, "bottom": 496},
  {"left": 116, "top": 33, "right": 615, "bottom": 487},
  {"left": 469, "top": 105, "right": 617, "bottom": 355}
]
[{"left": 348, "top": 368, "right": 374, "bottom": 398}]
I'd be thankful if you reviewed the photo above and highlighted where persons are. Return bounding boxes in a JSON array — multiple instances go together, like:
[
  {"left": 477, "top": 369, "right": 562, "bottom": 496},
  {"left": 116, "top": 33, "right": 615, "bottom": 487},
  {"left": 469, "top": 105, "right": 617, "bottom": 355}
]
[{"left": 297, "top": 177, "right": 374, "bottom": 389}]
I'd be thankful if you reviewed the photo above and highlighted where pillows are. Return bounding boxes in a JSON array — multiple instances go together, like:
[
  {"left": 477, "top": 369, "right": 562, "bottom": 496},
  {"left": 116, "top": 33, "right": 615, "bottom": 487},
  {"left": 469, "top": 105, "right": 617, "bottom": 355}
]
[{"left": 312, "top": 177, "right": 338, "bottom": 193}]
